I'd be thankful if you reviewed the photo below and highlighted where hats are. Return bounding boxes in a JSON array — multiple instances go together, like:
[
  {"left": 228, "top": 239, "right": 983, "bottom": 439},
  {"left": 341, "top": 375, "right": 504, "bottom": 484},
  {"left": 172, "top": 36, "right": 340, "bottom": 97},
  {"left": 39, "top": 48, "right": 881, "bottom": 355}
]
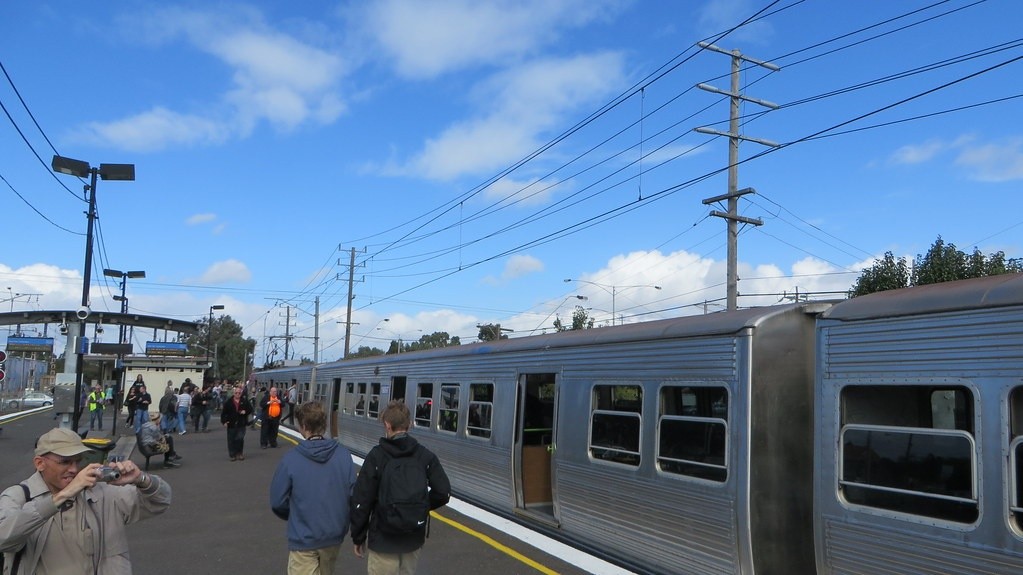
[
  {"left": 150, "top": 412, "right": 163, "bottom": 422},
  {"left": 35, "top": 427, "right": 96, "bottom": 457}
]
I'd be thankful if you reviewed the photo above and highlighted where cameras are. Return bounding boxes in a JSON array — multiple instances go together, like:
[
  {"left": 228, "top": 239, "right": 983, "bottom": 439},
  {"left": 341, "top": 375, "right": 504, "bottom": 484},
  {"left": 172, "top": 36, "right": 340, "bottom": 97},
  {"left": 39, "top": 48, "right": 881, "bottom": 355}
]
[{"left": 93, "top": 467, "right": 121, "bottom": 482}]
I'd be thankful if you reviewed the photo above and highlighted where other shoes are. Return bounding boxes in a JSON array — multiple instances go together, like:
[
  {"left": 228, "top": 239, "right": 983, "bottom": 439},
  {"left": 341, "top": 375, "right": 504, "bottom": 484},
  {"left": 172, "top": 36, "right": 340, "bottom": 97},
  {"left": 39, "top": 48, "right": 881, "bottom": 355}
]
[
  {"left": 178, "top": 430, "right": 187, "bottom": 436},
  {"left": 125, "top": 422, "right": 134, "bottom": 428},
  {"left": 262, "top": 444, "right": 268, "bottom": 449},
  {"left": 230, "top": 453, "right": 245, "bottom": 461},
  {"left": 163, "top": 460, "right": 183, "bottom": 469},
  {"left": 168, "top": 454, "right": 183, "bottom": 462}
]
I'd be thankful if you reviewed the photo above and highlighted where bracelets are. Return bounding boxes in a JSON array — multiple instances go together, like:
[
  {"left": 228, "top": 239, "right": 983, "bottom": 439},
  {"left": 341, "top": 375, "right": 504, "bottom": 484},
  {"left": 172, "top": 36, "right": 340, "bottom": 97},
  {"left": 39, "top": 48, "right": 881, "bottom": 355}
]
[{"left": 138, "top": 472, "right": 146, "bottom": 486}]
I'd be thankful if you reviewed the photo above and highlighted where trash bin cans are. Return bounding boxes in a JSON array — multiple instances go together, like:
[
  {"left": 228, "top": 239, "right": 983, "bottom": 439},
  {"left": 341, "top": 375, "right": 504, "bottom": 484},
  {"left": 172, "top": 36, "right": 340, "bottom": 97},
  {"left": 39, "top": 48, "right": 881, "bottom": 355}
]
[{"left": 77, "top": 437, "right": 116, "bottom": 468}]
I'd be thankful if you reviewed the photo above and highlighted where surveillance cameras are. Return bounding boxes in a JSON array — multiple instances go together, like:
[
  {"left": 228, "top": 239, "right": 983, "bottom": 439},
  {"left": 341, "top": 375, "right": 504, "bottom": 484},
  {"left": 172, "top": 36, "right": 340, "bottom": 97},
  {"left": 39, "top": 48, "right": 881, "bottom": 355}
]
[{"left": 77, "top": 307, "right": 90, "bottom": 319}]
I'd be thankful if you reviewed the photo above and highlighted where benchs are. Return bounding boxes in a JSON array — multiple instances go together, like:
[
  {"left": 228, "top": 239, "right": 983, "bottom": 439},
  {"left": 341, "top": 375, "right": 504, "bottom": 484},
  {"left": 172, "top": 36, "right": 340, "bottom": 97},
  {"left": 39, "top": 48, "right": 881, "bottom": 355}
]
[{"left": 135, "top": 419, "right": 170, "bottom": 470}]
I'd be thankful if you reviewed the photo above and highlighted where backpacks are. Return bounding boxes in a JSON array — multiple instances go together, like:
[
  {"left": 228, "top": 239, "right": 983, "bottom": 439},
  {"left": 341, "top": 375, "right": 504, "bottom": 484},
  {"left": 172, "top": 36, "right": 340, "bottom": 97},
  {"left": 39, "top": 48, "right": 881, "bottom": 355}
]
[
  {"left": 371, "top": 444, "right": 431, "bottom": 543},
  {"left": 283, "top": 388, "right": 293, "bottom": 403}
]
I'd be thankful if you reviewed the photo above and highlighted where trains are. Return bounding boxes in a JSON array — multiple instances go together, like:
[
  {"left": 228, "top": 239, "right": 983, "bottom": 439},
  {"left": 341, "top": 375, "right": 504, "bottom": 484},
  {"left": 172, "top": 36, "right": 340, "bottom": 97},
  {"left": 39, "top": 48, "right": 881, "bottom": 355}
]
[{"left": 246, "top": 272, "right": 1023, "bottom": 574}]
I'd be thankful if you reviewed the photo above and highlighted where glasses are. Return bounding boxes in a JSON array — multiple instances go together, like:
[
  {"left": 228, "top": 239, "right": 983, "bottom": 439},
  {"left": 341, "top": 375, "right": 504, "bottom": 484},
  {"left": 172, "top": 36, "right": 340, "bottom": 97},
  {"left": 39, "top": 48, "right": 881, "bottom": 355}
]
[{"left": 44, "top": 456, "right": 84, "bottom": 466}]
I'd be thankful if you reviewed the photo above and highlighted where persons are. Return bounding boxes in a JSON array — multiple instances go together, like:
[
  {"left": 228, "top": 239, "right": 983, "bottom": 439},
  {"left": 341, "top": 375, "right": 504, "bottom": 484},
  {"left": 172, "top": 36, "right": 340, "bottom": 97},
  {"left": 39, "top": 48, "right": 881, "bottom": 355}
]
[
  {"left": 351, "top": 400, "right": 451, "bottom": 575},
  {"left": 270, "top": 402, "right": 357, "bottom": 575},
  {"left": 0, "top": 428, "right": 172, "bottom": 575},
  {"left": 87, "top": 377, "right": 297, "bottom": 468}
]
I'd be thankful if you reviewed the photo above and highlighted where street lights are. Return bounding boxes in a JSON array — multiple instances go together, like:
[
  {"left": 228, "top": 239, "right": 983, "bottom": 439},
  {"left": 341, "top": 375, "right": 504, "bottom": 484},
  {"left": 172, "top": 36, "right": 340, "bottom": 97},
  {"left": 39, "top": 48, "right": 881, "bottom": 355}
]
[
  {"left": 113, "top": 295, "right": 129, "bottom": 411},
  {"left": 563, "top": 278, "right": 662, "bottom": 327},
  {"left": 376, "top": 328, "right": 422, "bottom": 354},
  {"left": 207, "top": 305, "right": 224, "bottom": 365},
  {"left": 103, "top": 268, "right": 146, "bottom": 437},
  {"left": 50, "top": 153, "right": 137, "bottom": 434},
  {"left": 344, "top": 319, "right": 390, "bottom": 355},
  {"left": 7, "top": 287, "right": 20, "bottom": 336}
]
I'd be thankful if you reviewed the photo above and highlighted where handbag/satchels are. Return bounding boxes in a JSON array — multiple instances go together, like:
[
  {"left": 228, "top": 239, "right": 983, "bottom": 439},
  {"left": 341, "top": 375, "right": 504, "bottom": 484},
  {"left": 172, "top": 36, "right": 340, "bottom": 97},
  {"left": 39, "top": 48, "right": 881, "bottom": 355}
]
[
  {"left": 154, "top": 439, "right": 170, "bottom": 453},
  {"left": 123, "top": 395, "right": 131, "bottom": 406}
]
